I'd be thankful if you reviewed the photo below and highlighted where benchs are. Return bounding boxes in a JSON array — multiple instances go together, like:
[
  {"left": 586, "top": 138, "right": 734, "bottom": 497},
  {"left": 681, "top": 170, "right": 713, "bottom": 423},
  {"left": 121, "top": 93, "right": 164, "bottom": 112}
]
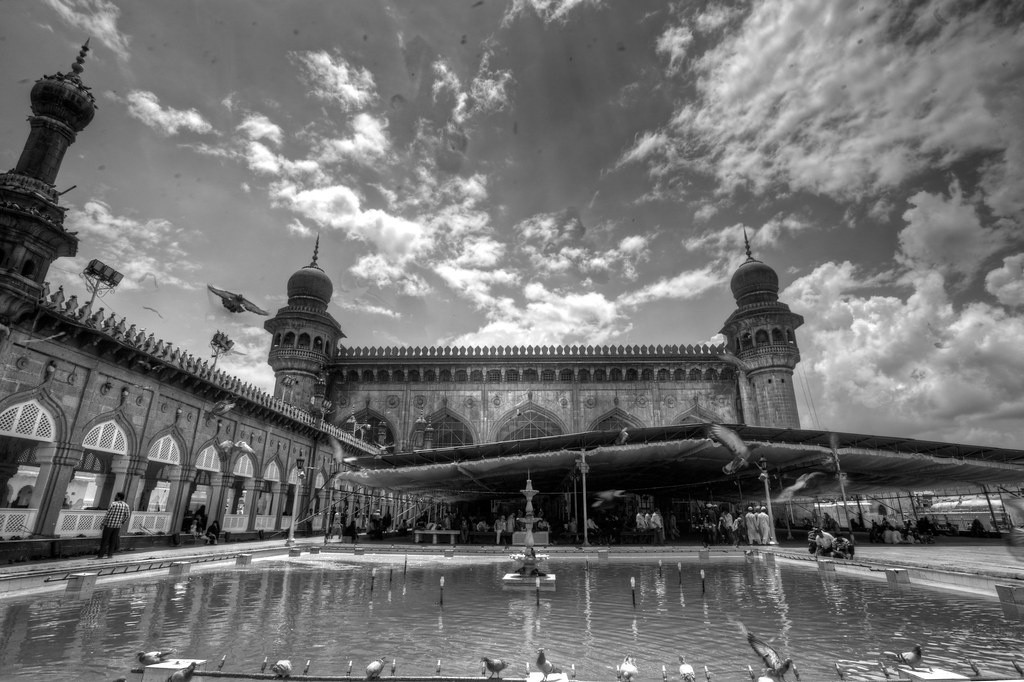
[
  {"left": 0, "top": 529, "right": 327, "bottom": 566},
  {"left": 415, "top": 530, "right": 461, "bottom": 545},
  {"left": 467, "top": 532, "right": 658, "bottom": 545}
]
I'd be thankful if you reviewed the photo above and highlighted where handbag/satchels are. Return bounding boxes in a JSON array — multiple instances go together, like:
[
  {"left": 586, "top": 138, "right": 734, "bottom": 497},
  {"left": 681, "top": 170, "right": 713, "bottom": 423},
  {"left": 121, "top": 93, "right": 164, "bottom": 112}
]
[{"left": 214, "top": 536, "right": 217, "bottom": 544}]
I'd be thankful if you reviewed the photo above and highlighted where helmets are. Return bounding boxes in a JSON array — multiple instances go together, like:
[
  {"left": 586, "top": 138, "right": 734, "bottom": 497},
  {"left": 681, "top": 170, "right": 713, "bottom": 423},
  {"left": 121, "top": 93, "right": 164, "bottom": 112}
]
[
  {"left": 748, "top": 506, "right": 752, "bottom": 510},
  {"left": 755, "top": 506, "right": 760, "bottom": 510},
  {"left": 760, "top": 506, "right": 766, "bottom": 510}
]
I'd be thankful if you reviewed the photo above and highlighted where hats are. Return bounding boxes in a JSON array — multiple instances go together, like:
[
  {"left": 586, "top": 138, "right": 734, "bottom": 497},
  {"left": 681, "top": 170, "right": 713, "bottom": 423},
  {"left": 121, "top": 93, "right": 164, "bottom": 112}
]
[{"left": 814, "top": 529, "right": 821, "bottom": 533}]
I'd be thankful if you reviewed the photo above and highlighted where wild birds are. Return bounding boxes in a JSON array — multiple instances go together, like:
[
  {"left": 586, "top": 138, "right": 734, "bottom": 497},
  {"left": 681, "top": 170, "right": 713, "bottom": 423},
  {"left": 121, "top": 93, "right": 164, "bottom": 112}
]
[
  {"left": 166, "top": 662, "right": 197, "bottom": 682},
  {"left": 143, "top": 306, "right": 164, "bottom": 319},
  {"left": 272, "top": 660, "right": 294, "bottom": 681},
  {"left": 747, "top": 632, "right": 792, "bottom": 682},
  {"left": 136, "top": 650, "right": 173, "bottom": 666},
  {"left": 366, "top": 657, "right": 388, "bottom": 680},
  {"left": 619, "top": 653, "right": 638, "bottom": 682},
  {"left": 207, "top": 284, "right": 271, "bottom": 317},
  {"left": 883, "top": 643, "right": 923, "bottom": 672},
  {"left": 535, "top": 648, "right": 562, "bottom": 682},
  {"left": 138, "top": 272, "right": 159, "bottom": 289},
  {"left": 480, "top": 657, "right": 512, "bottom": 681},
  {"left": 678, "top": 656, "right": 696, "bottom": 682}
]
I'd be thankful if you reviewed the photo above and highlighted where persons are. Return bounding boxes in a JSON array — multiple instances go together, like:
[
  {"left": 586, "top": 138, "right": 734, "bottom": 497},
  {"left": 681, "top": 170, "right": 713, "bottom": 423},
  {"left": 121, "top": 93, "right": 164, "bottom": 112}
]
[
  {"left": 305, "top": 508, "right": 314, "bottom": 536},
  {"left": 696, "top": 501, "right": 795, "bottom": 545},
  {"left": 94, "top": 492, "right": 130, "bottom": 559},
  {"left": 803, "top": 512, "right": 855, "bottom": 560},
  {"left": 869, "top": 516, "right": 958, "bottom": 544},
  {"left": 190, "top": 520, "right": 220, "bottom": 545},
  {"left": 324, "top": 507, "right": 680, "bottom": 546}
]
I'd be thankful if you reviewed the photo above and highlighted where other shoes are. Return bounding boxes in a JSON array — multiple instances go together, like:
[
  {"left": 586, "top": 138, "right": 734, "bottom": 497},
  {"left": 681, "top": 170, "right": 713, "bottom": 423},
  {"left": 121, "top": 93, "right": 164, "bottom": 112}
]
[
  {"left": 95, "top": 556, "right": 102, "bottom": 559},
  {"left": 107, "top": 556, "right": 112, "bottom": 558}
]
